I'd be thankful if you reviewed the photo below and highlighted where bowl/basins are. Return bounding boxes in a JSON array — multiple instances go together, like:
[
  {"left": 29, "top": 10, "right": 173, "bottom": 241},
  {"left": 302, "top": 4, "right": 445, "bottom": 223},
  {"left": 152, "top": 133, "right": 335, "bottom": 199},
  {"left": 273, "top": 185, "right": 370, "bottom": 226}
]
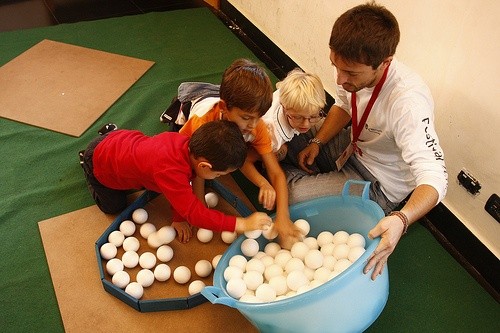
[{"left": 201, "top": 180, "right": 389, "bottom": 333}]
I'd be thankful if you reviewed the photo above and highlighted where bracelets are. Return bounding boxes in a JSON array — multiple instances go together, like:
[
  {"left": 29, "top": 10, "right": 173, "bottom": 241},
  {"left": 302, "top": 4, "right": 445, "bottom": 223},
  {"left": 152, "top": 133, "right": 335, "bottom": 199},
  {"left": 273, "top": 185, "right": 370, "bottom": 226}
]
[{"left": 390, "top": 211, "right": 408, "bottom": 235}]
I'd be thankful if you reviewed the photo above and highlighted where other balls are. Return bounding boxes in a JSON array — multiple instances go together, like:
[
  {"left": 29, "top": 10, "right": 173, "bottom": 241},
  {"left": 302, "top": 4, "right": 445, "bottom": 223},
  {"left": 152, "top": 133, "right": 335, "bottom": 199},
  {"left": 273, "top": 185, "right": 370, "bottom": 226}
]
[{"left": 100, "top": 182, "right": 366, "bottom": 305}]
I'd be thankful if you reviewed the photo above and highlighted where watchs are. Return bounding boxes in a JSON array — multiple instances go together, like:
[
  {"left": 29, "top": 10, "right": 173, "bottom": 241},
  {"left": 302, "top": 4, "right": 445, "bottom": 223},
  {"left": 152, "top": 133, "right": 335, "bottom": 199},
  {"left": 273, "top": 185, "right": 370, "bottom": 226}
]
[{"left": 307, "top": 139, "right": 322, "bottom": 149}]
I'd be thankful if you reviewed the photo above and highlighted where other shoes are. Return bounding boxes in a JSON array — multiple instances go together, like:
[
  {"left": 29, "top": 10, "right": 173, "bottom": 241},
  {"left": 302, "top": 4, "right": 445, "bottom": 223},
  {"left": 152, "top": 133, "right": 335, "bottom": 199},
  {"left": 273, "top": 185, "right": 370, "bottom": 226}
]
[
  {"left": 99, "top": 123, "right": 117, "bottom": 136},
  {"left": 79, "top": 150, "right": 87, "bottom": 168}
]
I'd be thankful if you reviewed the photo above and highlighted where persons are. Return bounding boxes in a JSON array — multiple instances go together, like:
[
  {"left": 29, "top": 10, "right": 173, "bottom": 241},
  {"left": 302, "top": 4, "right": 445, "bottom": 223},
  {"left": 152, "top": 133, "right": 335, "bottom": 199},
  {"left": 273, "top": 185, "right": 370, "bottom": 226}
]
[
  {"left": 241, "top": 67, "right": 326, "bottom": 211},
  {"left": 288, "top": 3, "right": 447, "bottom": 280},
  {"left": 157, "top": 57, "right": 299, "bottom": 248},
  {"left": 78, "top": 120, "right": 273, "bottom": 243}
]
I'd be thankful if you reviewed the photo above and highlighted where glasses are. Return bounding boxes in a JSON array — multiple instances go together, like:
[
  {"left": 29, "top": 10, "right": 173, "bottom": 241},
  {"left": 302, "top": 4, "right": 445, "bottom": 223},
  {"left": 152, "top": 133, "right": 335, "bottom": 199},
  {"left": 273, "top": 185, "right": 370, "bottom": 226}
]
[{"left": 286, "top": 109, "right": 323, "bottom": 123}]
